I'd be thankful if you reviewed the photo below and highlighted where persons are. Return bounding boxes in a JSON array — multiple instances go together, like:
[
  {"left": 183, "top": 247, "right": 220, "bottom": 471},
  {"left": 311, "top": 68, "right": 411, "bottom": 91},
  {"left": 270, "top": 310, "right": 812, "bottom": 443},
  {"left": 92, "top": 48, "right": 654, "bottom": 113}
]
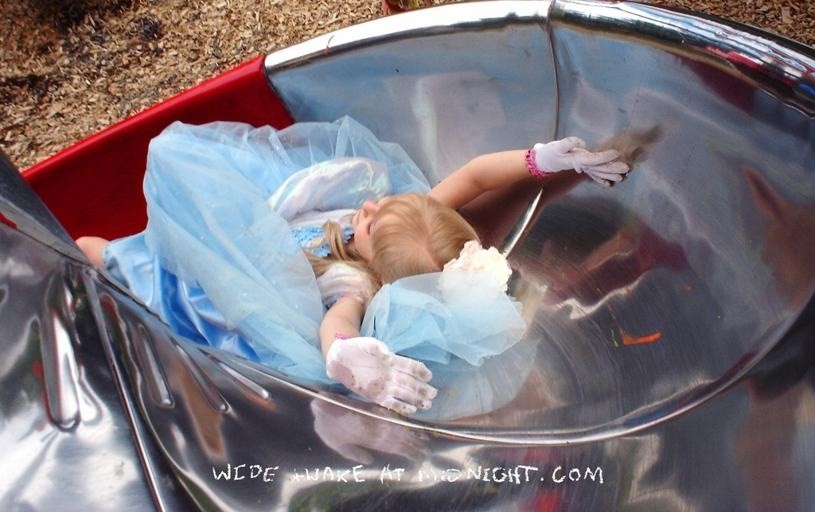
[
  {"left": 73, "top": 135, "right": 631, "bottom": 417},
  {"left": 310, "top": 390, "right": 431, "bottom": 466}
]
